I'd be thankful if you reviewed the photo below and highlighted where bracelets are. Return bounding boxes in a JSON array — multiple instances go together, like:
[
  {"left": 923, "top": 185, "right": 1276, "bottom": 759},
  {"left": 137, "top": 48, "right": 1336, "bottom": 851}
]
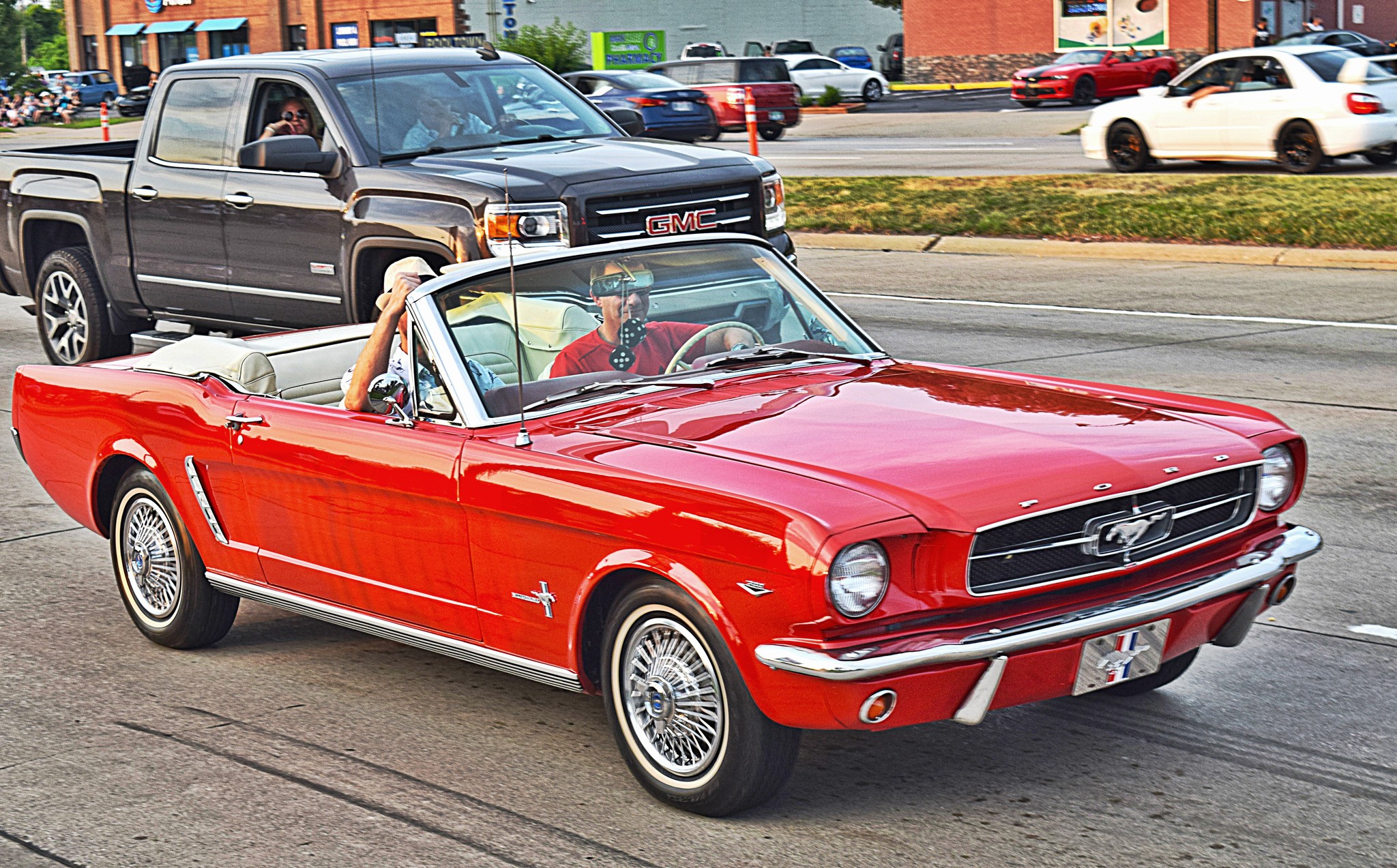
[{"left": 265, "top": 123, "right": 279, "bottom": 133}]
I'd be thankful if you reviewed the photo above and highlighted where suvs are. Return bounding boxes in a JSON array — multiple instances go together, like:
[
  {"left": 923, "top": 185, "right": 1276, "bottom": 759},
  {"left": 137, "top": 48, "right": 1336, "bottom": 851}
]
[
  {"left": 743, "top": 40, "right": 820, "bottom": 58},
  {"left": 877, "top": 33, "right": 904, "bottom": 81},
  {"left": 677, "top": 42, "right": 735, "bottom": 64}
]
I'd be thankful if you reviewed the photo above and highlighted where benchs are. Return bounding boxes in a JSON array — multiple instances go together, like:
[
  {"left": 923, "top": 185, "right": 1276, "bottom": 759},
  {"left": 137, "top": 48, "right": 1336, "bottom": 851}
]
[{"left": 137, "top": 323, "right": 532, "bottom": 406}]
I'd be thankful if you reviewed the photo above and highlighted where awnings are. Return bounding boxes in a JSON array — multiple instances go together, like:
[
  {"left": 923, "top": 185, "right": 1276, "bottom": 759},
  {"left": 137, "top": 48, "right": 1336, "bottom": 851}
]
[
  {"left": 193, "top": 17, "right": 249, "bottom": 32},
  {"left": 104, "top": 23, "right": 146, "bottom": 35},
  {"left": 142, "top": 20, "right": 195, "bottom": 34}
]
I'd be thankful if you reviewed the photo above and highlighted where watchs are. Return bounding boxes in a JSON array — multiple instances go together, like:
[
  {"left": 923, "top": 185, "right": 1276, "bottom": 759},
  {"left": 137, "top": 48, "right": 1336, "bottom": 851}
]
[{"left": 730, "top": 343, "right": 752, "bottom": 351}]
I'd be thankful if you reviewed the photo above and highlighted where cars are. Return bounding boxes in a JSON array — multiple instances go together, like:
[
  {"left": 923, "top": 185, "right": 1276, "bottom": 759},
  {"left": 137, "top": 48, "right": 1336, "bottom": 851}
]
[
  {"left": 111, "top": 64, "right": 161, "bottom": 117},
  {"left": 1080, "top": 46, "right": 1397, "bottom": 175},
  {"left": 829, "top": 46, "right": 874, "bottom": 72},
  {"left": 778, "top": 54, "right": 891, "bottom": 100},
  {"left": 501, "top": 69, "right": 719, "bottom": 143},
  {"left": 1264, "top": 30, "right": 1391, "bottom": 83},
  {"left": 647, "top": 56, "right": 805, "bottom": 143},
  {"left": 49, "top": 70, "right": 120, "bottom": 112},
  {"left": 37, "top": 70, "right": 71, "bottom": 91}
]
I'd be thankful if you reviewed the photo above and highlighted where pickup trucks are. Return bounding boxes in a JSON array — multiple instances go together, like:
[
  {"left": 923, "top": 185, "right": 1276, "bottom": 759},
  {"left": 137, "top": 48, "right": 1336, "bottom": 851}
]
[{"left": 0, "top": 46, "right": 799, "bottom": 353}]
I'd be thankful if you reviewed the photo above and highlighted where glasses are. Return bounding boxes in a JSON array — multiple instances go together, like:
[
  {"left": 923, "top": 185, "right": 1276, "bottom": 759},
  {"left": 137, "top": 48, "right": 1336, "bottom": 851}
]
[
  {"left": 281, "top": 109, "right": 310, "bottom": 122},
  {"left": 618, "top": 285, "right": 654, "bottom": 298},
  {"left": 420, "top": 97, "right": 452, "bottom": 107}
]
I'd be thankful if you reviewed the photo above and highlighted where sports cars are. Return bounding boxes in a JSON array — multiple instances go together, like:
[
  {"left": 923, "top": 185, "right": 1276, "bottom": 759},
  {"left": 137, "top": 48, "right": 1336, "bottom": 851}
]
[
  {"left": 1010, "top": 49, "right": 1180, "bottom": 108},
  {"left": 11, "top": 234, "right": 1325, "bottom": 820}
]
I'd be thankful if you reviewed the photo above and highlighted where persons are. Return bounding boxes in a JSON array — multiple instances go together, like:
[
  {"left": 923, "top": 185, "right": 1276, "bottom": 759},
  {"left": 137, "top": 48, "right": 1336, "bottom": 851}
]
[
  {"left": 1124, "top": 46, "right": 1157, "bottom": 59},
  {"left": 255, "top": 96, "right": 323, "bottom": 166},
  {"left": 0, "top": 66, "right": 85, "bottom": 128},
  {"left": 1248, "top": 18, "right": 1272, "bottom": 49},
  {"left": 548, "top": 257, "right": 754, "bottom": 378},
  {"left": 403, "top": 77, "right": 515, "bottom": 151},
  {"left": 1186, "top": 60, "right": 1247, "bottom": 108},
  {"left": 339, "top": 256, "right": 507, "bottom": 417},
  {"left": 1302, "top": 15, "right": 1324, "bottom": 33},
  {"left": 148, "top": 72, "right": 159, "bottom": 97}
]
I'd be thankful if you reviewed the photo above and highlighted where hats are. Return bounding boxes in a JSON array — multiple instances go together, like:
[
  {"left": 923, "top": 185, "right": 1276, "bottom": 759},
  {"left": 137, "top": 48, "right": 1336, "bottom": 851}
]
[
  {"left": 38, "top": 91, "right": 50, "bottom": 97},
  {"left": 375, "top": 256, "right": 448, "bottom": 311}
]
